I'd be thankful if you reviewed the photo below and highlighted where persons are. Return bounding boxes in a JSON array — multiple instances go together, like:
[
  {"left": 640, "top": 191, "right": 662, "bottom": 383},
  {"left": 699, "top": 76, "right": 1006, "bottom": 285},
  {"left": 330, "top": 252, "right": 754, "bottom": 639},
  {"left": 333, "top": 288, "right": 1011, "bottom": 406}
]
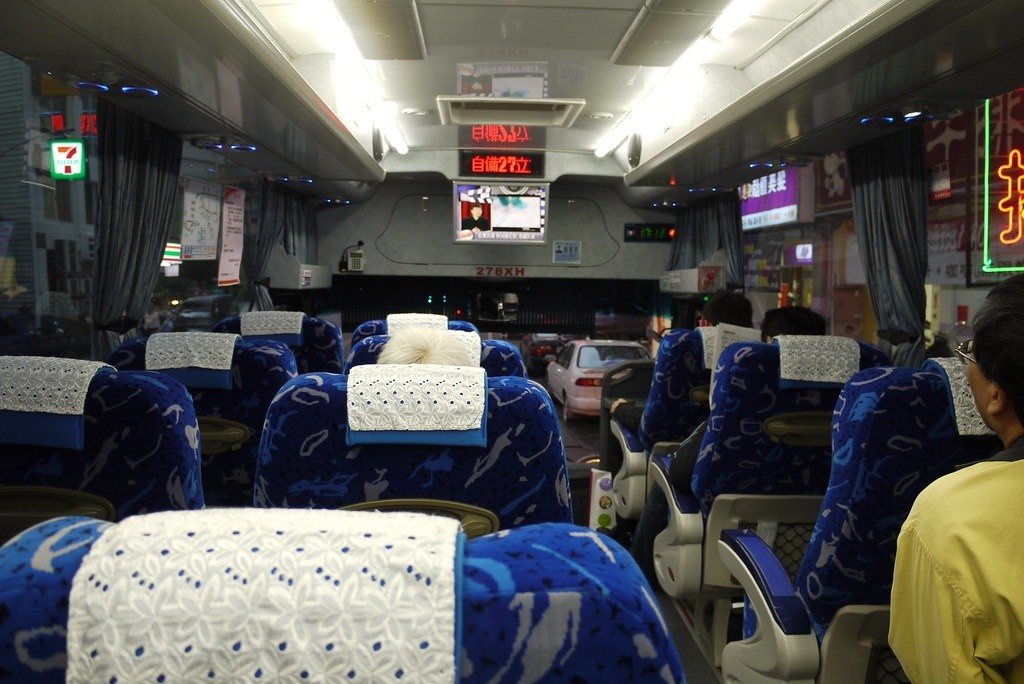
[
  {"left": 461, "top": 203, "right": 490, "bottom": 232},
  {"left": 631, "top": 305, "right": 828, "bottom": 590},
  {"left": 375, "top": 328, "right": 472, "bottom": 370},
  {"left": 606, "top": 288, "right": 753, "bottom": 435},
  {"left": 556, "top": 245, "right": 563, "bottom": 253},
  {"left": 888, "top": 273, "right": 1024, "bottom": 684}
]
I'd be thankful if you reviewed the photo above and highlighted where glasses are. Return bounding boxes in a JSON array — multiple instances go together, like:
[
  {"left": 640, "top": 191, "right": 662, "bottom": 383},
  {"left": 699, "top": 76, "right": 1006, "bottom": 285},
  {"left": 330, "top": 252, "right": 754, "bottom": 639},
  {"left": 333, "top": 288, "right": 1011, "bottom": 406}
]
[{"left": 955, "top": 341, "right": 978, "bottom": 364}]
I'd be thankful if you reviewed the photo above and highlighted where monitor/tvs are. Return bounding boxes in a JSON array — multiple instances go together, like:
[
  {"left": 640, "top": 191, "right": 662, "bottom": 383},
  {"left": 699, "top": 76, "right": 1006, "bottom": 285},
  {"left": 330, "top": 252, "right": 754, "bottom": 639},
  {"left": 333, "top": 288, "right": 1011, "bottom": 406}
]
[{"left": 453, "top": 181, "right": 550, "bottom": 245}]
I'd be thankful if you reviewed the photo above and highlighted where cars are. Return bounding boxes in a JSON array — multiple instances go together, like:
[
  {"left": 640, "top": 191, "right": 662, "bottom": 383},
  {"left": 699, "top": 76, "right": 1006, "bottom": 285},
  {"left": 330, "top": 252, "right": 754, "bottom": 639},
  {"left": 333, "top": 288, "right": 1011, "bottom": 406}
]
[
  {"left": 519, "top": 333, "right": 591, "bottom": 372},
  {"left": 544, "top": 339, "right": 654, "bottom": 426}
]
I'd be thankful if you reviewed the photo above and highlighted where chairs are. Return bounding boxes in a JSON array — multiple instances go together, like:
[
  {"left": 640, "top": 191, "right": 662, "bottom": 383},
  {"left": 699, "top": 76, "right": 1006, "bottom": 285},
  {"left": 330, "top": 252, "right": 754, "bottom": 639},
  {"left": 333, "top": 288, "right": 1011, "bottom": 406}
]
[{"left": 0, "top": 312, "right": 1008, "bottom": 684}]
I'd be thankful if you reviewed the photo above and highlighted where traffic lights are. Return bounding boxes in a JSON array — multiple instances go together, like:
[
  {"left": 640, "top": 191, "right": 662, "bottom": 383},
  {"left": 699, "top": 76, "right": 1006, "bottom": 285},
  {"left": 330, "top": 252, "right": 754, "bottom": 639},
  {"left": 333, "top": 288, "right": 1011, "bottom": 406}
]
[
  {"left": 428, "top": 295, "right": 432, "bottom": 304},
  {"left": 456, "top": 309, "right": 461, "bottom": 315},
  {"left": 442, "top": 295, "right": 447, "bottom": 304}
]
